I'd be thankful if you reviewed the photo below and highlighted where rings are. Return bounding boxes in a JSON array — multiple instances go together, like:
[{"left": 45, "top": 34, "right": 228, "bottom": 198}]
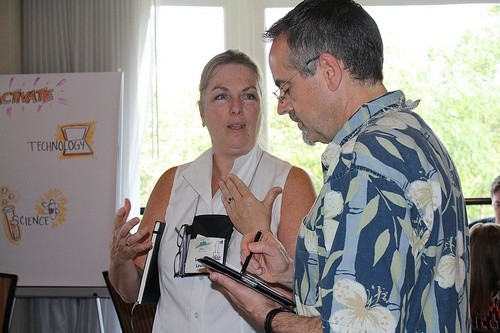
[
  {"left": 228, "top": 197, "right": 234, "bottom": 203},
  {"left": 124, "top": 239, "right": 131, "bottom": 246}
]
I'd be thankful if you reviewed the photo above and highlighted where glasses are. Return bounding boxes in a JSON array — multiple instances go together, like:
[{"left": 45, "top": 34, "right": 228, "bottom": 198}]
[
  {"left": 174, "top": 224, "right": 190, "bottom": 277},
  {"left": 273, "top": 56, "right": 321, "bottom": 105}
]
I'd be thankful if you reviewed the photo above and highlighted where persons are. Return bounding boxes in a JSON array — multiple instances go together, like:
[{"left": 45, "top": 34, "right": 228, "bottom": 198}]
[
  {"left": 108, "top": 49, "right": 318, "bottom": 333},
  {"left": 207, "top": 1, "right": 500, "bottom": 333}
]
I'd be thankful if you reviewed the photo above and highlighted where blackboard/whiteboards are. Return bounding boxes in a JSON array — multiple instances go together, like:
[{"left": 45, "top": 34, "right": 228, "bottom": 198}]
[{"left": 0, "top": 71, "right": 125, "bottom": 298}]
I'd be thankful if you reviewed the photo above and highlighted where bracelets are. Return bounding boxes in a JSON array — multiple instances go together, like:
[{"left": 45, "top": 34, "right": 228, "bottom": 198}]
[{"left": 264, "top": 307, "right": 297, "bottom": 333}]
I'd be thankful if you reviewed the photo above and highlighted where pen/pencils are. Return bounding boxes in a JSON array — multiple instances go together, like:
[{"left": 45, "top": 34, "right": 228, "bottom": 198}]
[{"left": 241, "top": 229, "right": 263, "bottom": 275}]
